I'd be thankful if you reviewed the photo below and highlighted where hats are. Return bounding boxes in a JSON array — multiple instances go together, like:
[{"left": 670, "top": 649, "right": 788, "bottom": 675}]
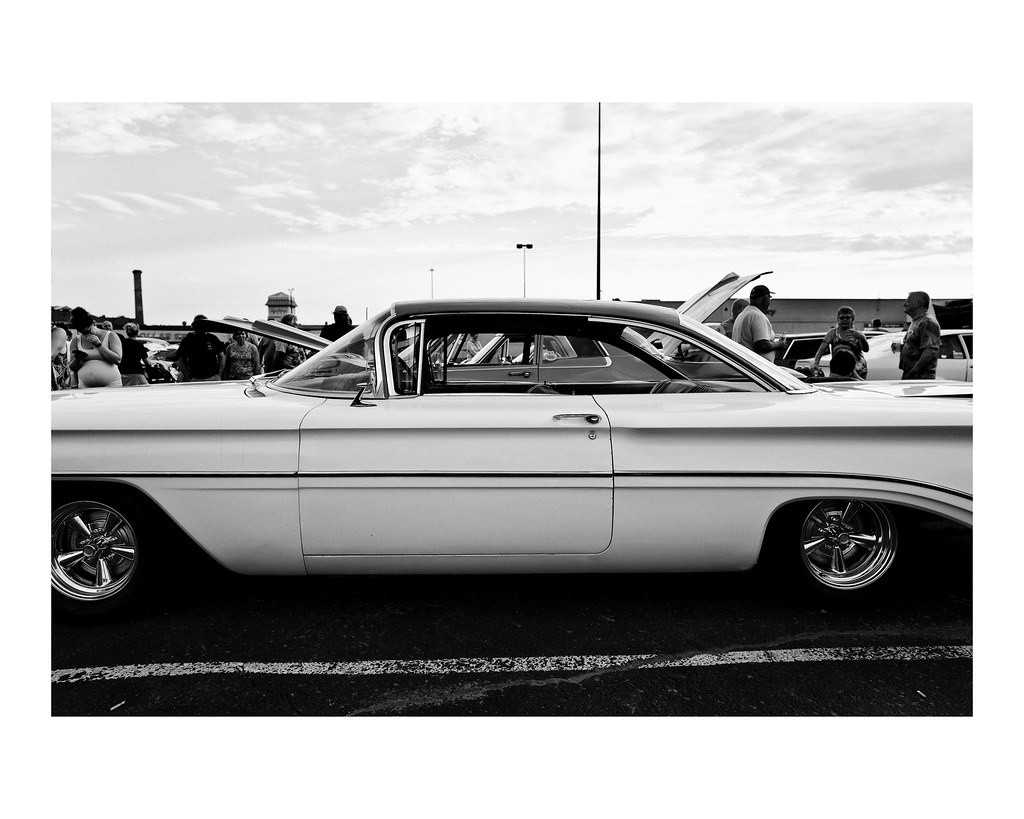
[
  {"left": 333, "top": 306, "right": 347, "bottom": 315},
  {"left": 750, "top": 285, "right": 775, "bottom": 296}
]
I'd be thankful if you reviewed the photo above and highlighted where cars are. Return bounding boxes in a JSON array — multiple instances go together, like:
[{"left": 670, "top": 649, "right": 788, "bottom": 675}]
[
  {"left": 775, "top": 331, "right": 889, "bottom": 370},
  {"left": 50, "top": 298, "right": 973, "bottom": 626},
  {"left": 703, "top": 323, "right": 721, "bottom": 330},
  {"left": 134, "top": 338, "right": 188, "bottom": 384},
  {"left": 372, "top": 326, "right": 692, "bottom": 387},
  {"left": 393, "top": 336, "right": 443, "bottom": 370},
  {"left": 793, "top": 328, "right": 973, "bottom": 383}
]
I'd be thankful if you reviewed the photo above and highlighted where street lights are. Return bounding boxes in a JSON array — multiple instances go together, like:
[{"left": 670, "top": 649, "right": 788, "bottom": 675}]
[
  {"left": 517, "top": 244, "right": 533, "bottom": 299},
  {"left": 288, "top": 288, "right": 295, "bottom": 313},
  {"left": 430, "top": 269, "right": 435, "bottom": 298}
]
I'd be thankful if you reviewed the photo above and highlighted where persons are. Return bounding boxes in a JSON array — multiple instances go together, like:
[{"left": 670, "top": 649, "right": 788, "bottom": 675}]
[
  {"left": 732, "top": 285, "right": 788, "bottom": 363},
  {"left": 466, "top": 333, "right": 482, "bottom": 362},
  {"left": 51, "top": 306, "right": 153, "bottom": 390},
  {"left": 814, "top": 306, "right": 869, "bottom": 379},
  {"left": 223, "top": 330, "right": 261, "bottom": 380},
  {"left": 307, "top": 305, "right": 365, "bottom": 360},
  {"left": 716, "top": 299, "right": 749, "bottom": 339},
  {"left": 258, "top": 314, "right": 303, "bottom": 377},
  {"left": 175, "top": 315, "right": 226, "bottom": 382},
  {"left": 891, "top": 292, "right": 940, "bottom": 380}
]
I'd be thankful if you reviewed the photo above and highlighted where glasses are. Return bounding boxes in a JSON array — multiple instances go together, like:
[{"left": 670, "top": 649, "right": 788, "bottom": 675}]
[{"left": 839, "top": 316, "right": 851, "bottom": 320}]
